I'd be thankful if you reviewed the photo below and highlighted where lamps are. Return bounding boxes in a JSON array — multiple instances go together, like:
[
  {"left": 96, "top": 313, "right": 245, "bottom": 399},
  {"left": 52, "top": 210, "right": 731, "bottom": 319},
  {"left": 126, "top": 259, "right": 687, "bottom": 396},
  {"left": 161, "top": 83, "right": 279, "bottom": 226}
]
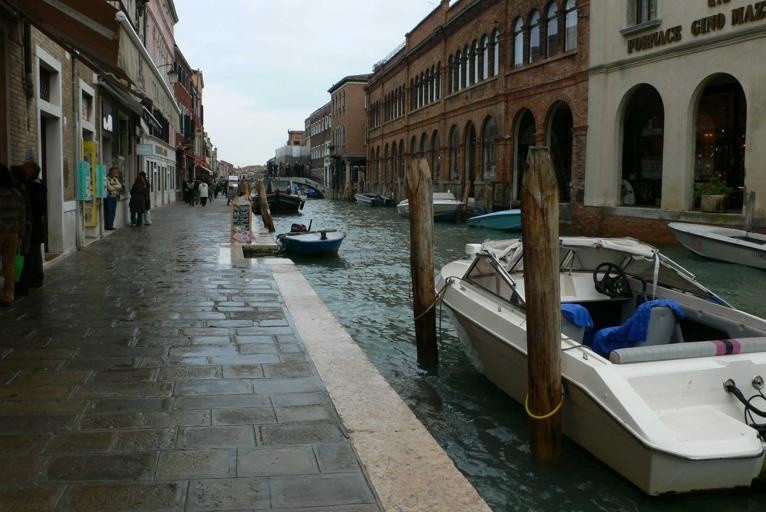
[{"left": 155, "top": 63, "right": 178, "bottom": 86}]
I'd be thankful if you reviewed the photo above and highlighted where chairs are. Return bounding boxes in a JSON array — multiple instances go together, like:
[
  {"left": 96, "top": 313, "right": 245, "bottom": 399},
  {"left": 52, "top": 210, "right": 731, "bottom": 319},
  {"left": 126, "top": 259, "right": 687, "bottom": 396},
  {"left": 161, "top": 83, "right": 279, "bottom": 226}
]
[
  {"left": 562, "top": 304, "right": 593, "bottom": 343},
  {"left": 594, "top": 300, "right": 683, "bottom": 357}
]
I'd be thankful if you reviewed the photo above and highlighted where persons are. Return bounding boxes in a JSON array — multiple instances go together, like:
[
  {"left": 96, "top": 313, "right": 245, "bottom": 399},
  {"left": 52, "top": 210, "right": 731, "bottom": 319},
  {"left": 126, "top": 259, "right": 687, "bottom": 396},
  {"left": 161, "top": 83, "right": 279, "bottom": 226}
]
[
  {"left": 16, "top": 160, "right": 47, "bottom": 287},
  {"left": 181, "top": 177, "right": 227, "bottom": 207},
  {"left": 140, "top": 171, "right": 152, "bottom": 225},
  {"left": 0, "top": 166, "right": 22, "bottom": 303},
  {"left": 129, "top": 175, "right": 145, "bottom": 226},
  {"left": 104, "top": 167, "right": 122, "bottom": 230},
  {"left": 263, "top": 159, "right": 315, "bottom": 180}
]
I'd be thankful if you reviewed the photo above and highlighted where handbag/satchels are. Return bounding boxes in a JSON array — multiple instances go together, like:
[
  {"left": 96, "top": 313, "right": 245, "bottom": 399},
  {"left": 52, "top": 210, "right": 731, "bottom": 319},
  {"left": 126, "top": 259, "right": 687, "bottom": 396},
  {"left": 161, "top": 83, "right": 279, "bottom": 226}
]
[{"left": 16, "top": 248, "right": 24, "bottom": 282}]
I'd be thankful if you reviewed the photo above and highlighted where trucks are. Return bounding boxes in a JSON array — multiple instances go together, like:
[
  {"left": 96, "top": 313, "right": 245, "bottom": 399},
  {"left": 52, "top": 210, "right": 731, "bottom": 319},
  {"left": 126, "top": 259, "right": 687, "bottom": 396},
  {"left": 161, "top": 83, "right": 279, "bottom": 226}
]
[{"left": 228, "top": 175, "right": 239, "bottom": 188}]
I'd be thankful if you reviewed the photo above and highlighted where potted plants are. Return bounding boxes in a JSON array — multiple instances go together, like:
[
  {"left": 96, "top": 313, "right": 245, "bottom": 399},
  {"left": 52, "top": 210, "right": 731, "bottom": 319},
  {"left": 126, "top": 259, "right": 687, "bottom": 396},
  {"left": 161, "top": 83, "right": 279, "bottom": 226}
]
[{"left": 693, "top": 172, "right": 732, "bottom": 212}]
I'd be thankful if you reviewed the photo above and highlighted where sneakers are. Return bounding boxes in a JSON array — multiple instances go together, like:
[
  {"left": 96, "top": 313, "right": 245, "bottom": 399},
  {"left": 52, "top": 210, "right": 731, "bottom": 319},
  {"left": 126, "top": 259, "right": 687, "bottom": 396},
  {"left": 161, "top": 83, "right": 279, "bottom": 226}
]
[
  {"left": 129, "top": 221, "right": 152, "bottom": 226},
  {"left": 106, "top": 228, "right": 116, "bottom": 230}
]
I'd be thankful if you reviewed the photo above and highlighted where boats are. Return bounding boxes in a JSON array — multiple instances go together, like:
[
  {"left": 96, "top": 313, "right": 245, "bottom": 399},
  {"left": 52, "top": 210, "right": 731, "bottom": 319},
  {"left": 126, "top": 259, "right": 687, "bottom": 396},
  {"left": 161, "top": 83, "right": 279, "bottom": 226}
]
[
  {"left": 396, "top": 191, "right": 467, "bottom": 221},
  {"left": 463, "top": 206, "right": 522, "bottom": 231},
  {"left": 429, "top": 230, "right": 766, "bottom": 498},
  {"left": 250, "top": 188, "right": 306, "bottom": 216},
  {"left": 353, "top": 192, "right": 394, "bottom": 208},
  {"left": 664, "top": 220, "right": 766, "bottom": 270},
  {"left": 278, "top": 218, "right": 347, "bottom": 255}
]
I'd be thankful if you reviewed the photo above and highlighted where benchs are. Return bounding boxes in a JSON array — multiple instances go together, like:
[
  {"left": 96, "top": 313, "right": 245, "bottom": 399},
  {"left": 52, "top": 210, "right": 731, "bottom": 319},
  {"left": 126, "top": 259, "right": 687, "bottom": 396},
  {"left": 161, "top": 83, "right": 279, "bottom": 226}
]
[{"left": 608, "top": 336, "right": 764, "bottom": 364}]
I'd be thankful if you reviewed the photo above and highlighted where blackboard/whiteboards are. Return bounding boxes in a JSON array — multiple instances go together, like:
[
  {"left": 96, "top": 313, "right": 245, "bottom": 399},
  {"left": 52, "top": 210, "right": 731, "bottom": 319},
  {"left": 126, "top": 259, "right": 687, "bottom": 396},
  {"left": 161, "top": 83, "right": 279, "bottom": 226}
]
[{"left": 230, "top": 202, "right": 252, "bottom": 243}]
